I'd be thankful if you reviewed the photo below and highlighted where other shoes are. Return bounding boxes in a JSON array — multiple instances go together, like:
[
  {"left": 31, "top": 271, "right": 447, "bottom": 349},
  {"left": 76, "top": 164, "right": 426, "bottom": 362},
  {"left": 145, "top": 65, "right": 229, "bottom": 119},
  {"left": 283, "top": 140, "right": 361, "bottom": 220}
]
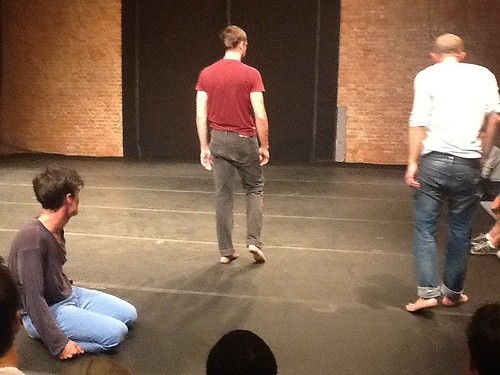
[
  {"left": 469, "top": 242, "right": 500, "bottom": 255},
  {"left": 470, "top": 233, "right": 491, "bottom": 246}
]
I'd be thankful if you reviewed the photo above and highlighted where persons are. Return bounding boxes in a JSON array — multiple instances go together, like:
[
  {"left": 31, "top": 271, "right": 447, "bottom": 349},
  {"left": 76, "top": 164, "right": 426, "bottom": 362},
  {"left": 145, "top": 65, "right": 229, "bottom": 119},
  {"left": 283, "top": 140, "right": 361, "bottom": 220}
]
[
  {"left": 195, "top": 25, "right": 269, "bottom": 264},
  {"left": 406, "top": 33, "right": 500, "bottom": 311},
  {"left": 0, "top": 256, "right": 26, "bottom": 375},
  {"left": 6, "top": 167, "right": 138, "bottom": 360},
  {"left": 469, "top": 115, "right": 500, "bottom": 254},
  {"left": 206, "top": 330, "right": 278, "bottom": 375}
]
